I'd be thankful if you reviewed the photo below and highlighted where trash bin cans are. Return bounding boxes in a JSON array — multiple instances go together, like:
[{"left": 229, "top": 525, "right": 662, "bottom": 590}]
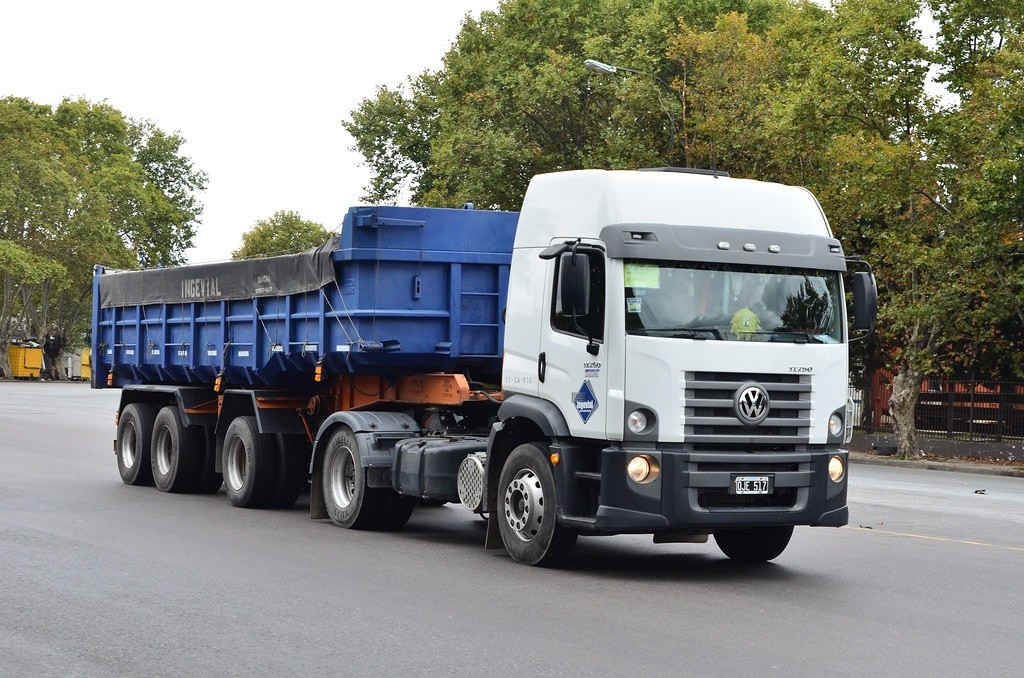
[
  {"left": 7, "top": 339, "right": 44, "bottom": 381},
  {"left": 62, "top": 345, "right": 91, "bottom": 381}
]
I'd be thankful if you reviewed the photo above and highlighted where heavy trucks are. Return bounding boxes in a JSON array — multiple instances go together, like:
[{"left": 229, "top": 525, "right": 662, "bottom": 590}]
[{"left": 89, "top": 162, "right": 880, "bottom": 568}]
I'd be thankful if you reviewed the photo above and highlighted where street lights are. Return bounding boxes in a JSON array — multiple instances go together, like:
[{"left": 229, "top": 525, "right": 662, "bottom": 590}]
[{"left": 583, "top": 57, "right": 675, "bottom": 166}]
